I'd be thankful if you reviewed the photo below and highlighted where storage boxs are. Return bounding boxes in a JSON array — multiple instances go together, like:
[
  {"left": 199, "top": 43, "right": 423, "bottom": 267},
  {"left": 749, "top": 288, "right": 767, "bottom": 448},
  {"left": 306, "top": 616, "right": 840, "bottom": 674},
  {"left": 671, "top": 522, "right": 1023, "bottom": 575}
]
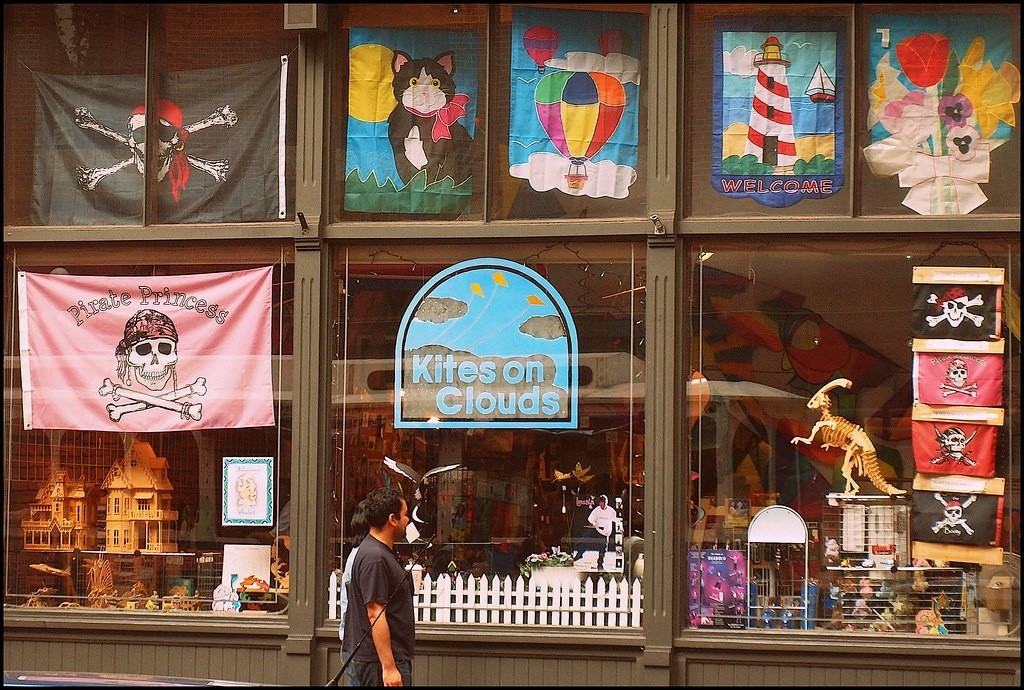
[
  {"left": 688, "top": 550, "right": 747, "bottom": 630},
  {"left": 985, "top": 577, "right": 1016, "bottom": 611}
]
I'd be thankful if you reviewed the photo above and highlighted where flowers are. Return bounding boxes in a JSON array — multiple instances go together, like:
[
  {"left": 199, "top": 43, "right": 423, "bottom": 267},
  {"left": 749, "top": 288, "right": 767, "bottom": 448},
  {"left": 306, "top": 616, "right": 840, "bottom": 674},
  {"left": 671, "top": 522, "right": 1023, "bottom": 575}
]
[{"left": 519, "top": 546, "right": 579, "bottom": 578}]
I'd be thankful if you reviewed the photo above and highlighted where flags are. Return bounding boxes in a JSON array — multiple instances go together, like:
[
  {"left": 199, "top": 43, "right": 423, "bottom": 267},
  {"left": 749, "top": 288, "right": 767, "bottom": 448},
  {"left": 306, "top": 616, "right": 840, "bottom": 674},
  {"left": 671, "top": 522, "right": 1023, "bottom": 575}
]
[
  {"left": 15, "top": 266, "right": 277, "bottom": 433},
  {"left": 28, "top": 54, "right": 289, "bottom": 225}
]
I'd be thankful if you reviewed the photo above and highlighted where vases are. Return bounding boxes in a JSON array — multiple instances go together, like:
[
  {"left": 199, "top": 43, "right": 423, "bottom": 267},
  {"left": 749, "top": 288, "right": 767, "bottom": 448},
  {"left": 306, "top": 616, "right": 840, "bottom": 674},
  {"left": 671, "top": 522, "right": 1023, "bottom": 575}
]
[{"left": 531, "top": 567, "right": 588, "bottom": 591}]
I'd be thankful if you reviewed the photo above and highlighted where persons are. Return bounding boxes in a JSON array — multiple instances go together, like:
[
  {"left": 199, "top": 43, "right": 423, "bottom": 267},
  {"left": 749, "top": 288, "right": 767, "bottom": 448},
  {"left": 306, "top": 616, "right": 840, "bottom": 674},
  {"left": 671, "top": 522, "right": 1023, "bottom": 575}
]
[
  {"left": 338, "top": 496, "right": 370, "bottom": 686},
  {"left": 349, "top": 488, "right": 417, "bottom": 687},
  {"left": 572, "top": 495, "right": 624, "bottom": 570}
]
